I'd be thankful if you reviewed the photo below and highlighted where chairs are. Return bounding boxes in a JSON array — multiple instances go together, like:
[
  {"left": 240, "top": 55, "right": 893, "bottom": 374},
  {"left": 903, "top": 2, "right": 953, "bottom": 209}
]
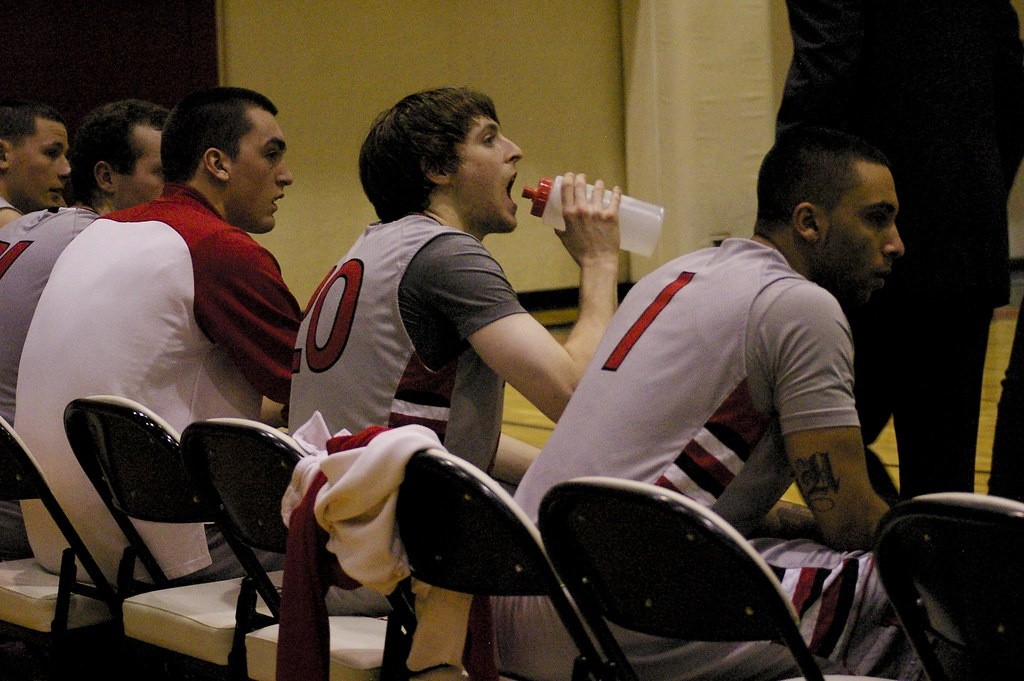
[
  {"left": 394, "top": 445, "right": 619, "bottom": 681},
  {"left": 0, "top": 413, "right": 146, "bottom": 681},
  {"left": 536, "top": 473, "right": 825, "bottom": 681},
  {"left": 872, "top": 491, "right": 1024, "bottom": 681},
  {"left": 63, "top": 395, "right": 286, "bottom": 681},
  {"left": 179, "top": 416, "right": 416, "bottom": 681}
]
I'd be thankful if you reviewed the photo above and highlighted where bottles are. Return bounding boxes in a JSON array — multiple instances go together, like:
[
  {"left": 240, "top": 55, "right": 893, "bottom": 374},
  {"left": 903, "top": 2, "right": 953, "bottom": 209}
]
[{"left": 522, "top": 177, "right": 663, "bottom": 260}]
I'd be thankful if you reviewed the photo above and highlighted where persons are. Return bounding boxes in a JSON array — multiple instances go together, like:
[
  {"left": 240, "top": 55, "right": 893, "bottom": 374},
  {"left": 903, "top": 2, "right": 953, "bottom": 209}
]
[
  {"left": 489, "top": 122, "right": 892, "bottom": 681},
  {"left": 287, "top": 86, "right": 622, "bottom": 619},
  {"left": 13, "top": 86, "right": 302, "bottom": 589},
  {"left": 0, "top": 98, "right": 170, "bottom": 429},
  {"left": 1, "top": 101, "right": 71, "bottom": 229},
  {"left": 774, "top": 0, "right": 1024, "bottom": 504}
]
